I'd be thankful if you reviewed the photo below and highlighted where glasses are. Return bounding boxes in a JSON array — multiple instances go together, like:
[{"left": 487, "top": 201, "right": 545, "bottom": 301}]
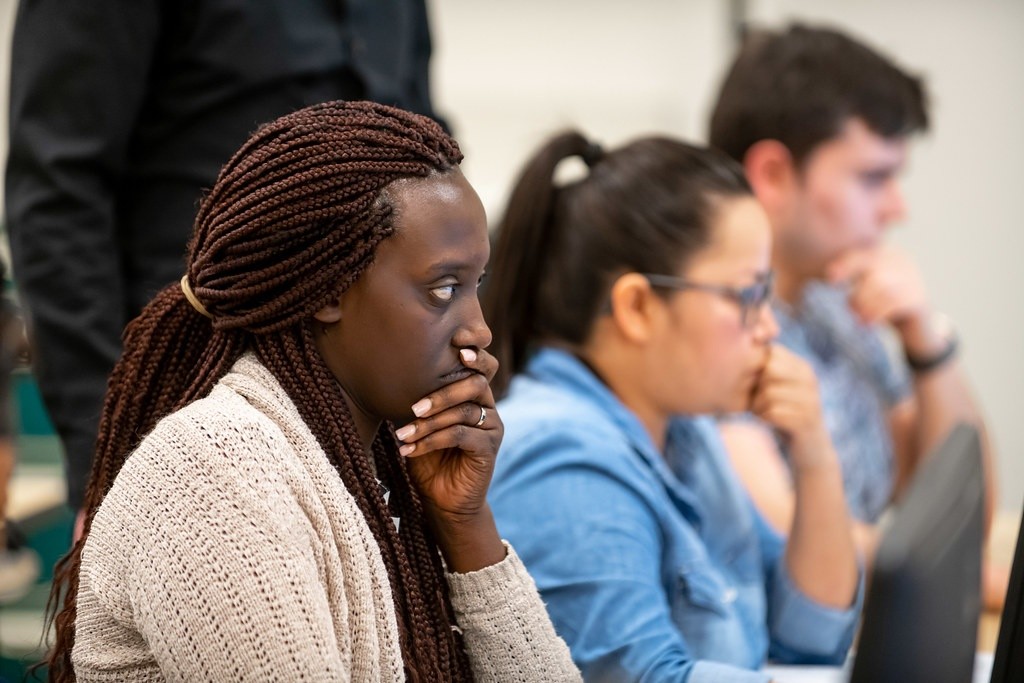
[{"left": 601, "top": 274, "right": 769, "bottom": 331}]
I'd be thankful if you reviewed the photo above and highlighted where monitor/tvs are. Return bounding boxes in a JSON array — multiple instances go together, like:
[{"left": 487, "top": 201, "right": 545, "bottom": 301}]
[
  {"left": 846, "top": 424, "right": 984, "bottom": 683},
  {"left": 990, "top": 508, "right": 1024, "bottom": 683}
]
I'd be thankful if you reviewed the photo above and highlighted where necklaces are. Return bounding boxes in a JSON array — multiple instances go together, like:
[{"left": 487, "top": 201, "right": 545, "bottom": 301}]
[{"left": 706, "top": 21, "right": 1011, "bottom": 612}]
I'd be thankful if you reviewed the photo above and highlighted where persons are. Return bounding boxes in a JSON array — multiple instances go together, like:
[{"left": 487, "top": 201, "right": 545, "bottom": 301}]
[
  {"left": 5, "top": 0, "right": 457, "bottom": 560},
  {"left": 480, "top": 130, "right": 866, "bottom": 683},
  {"left": 22, "top": 98, "right": 586, "bottom": 683}
]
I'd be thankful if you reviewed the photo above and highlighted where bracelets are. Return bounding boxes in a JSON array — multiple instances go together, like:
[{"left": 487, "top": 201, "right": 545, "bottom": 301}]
[{"left": 906, "top": 332, "right": 956, "bottom": 372}]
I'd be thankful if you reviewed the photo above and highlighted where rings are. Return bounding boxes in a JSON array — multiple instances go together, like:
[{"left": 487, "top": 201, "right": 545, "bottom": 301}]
[{"left": 476, "top": 406, "right": 486, "bottom": 427}]
